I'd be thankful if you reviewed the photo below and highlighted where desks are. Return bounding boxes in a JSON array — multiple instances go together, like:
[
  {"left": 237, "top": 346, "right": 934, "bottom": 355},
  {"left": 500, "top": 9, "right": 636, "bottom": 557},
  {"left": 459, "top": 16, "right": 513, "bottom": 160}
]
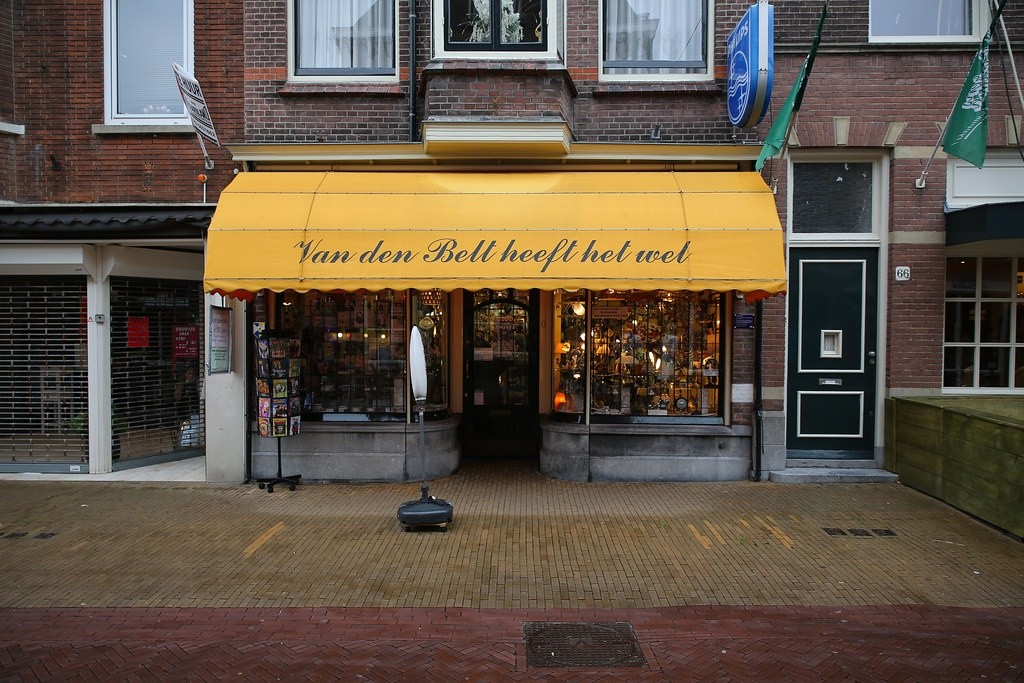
[{"left": 39, "top": 365, "right": 75, "bottom": 435}]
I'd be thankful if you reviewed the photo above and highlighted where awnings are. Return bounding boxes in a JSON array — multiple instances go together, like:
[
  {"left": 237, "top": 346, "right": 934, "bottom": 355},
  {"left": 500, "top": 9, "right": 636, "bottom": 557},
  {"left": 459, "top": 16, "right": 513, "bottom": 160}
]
[{"left": 203, "top": 172, "right": 789, "bottom": 303}]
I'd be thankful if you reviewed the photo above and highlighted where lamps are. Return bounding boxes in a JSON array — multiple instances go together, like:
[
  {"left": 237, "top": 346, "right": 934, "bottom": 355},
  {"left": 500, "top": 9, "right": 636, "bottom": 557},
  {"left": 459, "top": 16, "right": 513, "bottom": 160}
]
[{"left": 573, "top": 290, "right": 584, "bottom": 316}]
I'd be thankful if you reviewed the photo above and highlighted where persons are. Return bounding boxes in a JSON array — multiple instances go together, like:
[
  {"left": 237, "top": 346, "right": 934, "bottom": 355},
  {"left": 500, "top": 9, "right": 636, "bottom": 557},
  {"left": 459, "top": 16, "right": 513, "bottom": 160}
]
[
  {"left": 476, "top": 330, "right": 492, "bottom": 347},
  {"left": 272, "top": 341, "right": 276, "bottom": 355},
  {"left": 279, "top": 341, "right": 284, "bottom": 356},
  {"left": 615, "top": 346, "right": 641, "bottom": 405},
  {"left": 273, "top": 365, "right": 279, "bottom": 377},
  {"left": 291, "top": 420, "right": 299, "bottom": 434},
  {"left": 291, "top": 401, "right": 296, "bottom": 412},
  {"left": 276, "top": 380, "right": 285, "bottom": 392},
  {"left": 258, "top": 340, "right": 268, "bottom": 356},
  {"left": 291, "top": 340, "right": 297, "bottom": 358},
  {"left": 258, "top": 380, "right": 265, "bottom": 394},
  {"left": 259, "top": 422, "right": 268, "bottom": 434},
  {"left": 275, "top": 426, "right": 285, "bottom": 434},
  {"left": 515, "top": 325, "right": 527, "bottom": 352},
  {"left": 278, "top": 404, "right": 287, "bottom": 416}
]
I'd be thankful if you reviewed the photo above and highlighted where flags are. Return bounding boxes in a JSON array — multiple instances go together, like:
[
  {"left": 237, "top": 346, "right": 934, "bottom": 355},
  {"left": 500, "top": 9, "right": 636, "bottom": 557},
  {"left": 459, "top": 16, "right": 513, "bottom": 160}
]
[
  {"left": 754, "top": 55, "right": 808, "bottom": 171},
  {"left": 942, "top": 26, "right": 990, "bottom": 170}
]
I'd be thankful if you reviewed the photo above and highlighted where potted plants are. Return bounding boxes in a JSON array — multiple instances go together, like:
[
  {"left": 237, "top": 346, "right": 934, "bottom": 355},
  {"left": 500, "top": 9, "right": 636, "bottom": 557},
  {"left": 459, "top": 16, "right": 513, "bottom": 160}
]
[
  {"left": 70, "top": 400, "right": 123, "bottom": 463},
  {"left": 313, "top": 397, "right": 323, "bottom": 411}
]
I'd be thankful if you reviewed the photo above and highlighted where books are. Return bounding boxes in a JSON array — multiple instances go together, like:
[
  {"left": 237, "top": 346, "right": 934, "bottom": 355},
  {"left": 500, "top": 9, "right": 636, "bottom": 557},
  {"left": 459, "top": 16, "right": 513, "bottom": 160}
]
[{"left": 258, "top": 338, "right": 300, "bottom": 437}]
